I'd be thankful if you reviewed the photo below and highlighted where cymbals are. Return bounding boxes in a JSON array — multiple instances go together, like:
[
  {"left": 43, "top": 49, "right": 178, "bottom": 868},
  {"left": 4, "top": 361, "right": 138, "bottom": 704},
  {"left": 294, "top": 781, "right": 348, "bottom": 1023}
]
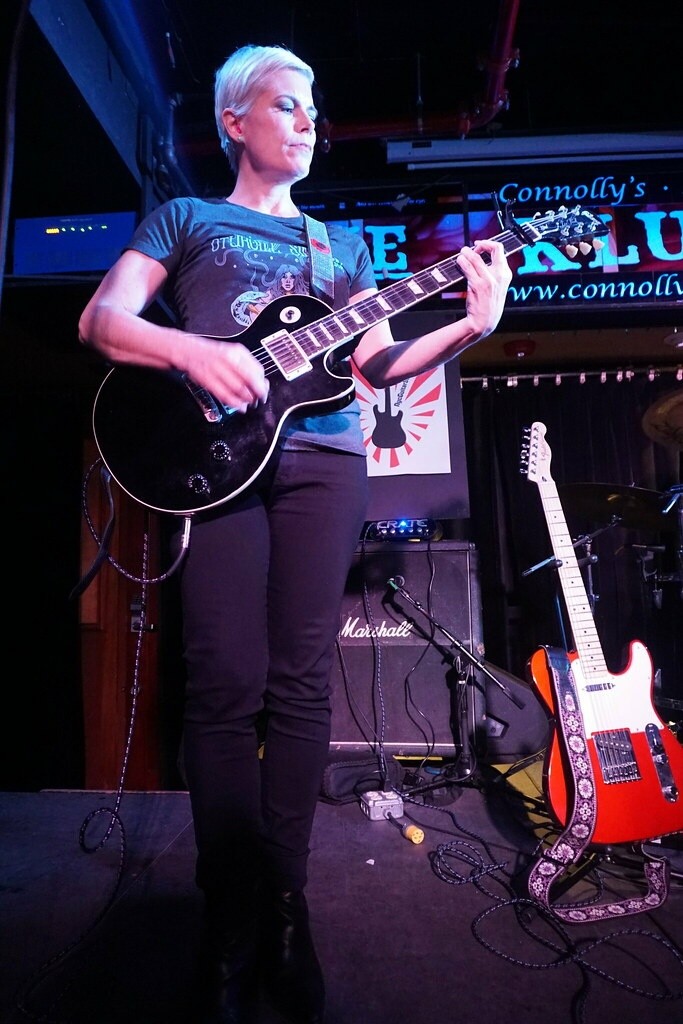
[
  {"left": 556, "top": 482, "right": 680, "bottom": 530},
  {"left": 641, "top": 387, "right": 683, "bottom": 447},
  {"left": 664, "top": 333, "right": 683, "bottom": 348}
]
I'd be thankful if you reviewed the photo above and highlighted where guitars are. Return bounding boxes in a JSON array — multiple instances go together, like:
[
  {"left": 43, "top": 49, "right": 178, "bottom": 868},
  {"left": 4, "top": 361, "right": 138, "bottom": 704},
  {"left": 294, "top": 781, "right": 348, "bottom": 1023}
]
[
  {"left": 90, "top": 204, "right": 607, "bottom": 519},
  {"left": 518, "top": 417, "right": 683, "bottom": 848}
]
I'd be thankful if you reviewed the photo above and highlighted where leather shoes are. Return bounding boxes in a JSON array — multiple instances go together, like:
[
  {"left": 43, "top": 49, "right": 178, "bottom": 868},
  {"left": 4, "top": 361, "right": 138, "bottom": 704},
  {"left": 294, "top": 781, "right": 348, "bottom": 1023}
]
[
  {"left": 256, "top": 888, "right": 326, "bottom": 1024},
  {"left": 207, "top": 935, "right": 258, "bottom": 1024}
]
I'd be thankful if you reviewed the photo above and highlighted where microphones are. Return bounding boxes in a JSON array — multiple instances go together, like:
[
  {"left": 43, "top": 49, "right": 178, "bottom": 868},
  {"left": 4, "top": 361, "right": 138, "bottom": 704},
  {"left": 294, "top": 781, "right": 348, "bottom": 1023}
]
[{"left": 385, "top": 575, "right": 405, "bottom": 588}]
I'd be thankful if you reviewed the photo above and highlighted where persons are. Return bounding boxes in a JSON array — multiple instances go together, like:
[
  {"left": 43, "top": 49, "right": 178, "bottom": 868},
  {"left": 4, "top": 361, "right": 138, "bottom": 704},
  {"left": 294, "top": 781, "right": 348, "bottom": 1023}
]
[{"left": 72, "top": 39, "right": 515, "bottom": 1024}]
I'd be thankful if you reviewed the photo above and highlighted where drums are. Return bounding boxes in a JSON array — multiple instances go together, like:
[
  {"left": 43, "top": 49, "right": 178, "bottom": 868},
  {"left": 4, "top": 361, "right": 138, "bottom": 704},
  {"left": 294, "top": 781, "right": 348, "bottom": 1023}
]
[{"left": 647, "top": 482, "right": 683, "bottom": 585}]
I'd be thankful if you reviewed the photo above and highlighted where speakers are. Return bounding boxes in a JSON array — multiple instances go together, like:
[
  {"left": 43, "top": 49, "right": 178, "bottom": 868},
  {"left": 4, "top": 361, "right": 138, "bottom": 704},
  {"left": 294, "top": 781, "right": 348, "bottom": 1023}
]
[{"left": 320, "top": 542, "right": 487, "bottom": 759}]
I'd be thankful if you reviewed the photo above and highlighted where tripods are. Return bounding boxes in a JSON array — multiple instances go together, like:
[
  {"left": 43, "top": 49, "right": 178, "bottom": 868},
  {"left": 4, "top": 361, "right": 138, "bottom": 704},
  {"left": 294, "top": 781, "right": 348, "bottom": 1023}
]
[{"left": 396, "top": 587, "right": 527, "bottom": 802}]
